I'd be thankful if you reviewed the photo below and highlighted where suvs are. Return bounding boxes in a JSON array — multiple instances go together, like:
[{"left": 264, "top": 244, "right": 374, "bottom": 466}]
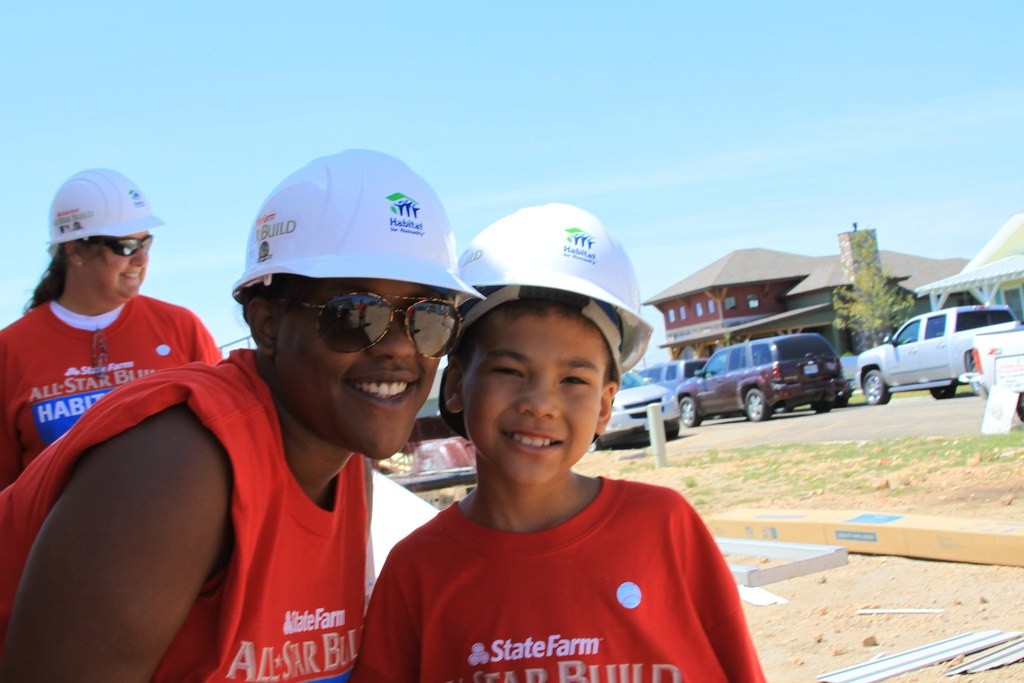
[
  {"left": 679, "top": 333, "right": 845, "bottom": 428},
  {"left": 640, "top": 358, "right": 708, "bottom": 392}
]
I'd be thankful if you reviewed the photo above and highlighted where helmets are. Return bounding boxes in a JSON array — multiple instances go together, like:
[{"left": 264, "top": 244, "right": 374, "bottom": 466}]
[
  {"left": 230, "top": 148, "right": 487, "bottom": 309},
  {"left": 455, "top": 202, "right": 652, "bottom": 374},
  {"left": 46, "top": 168, "right": 164, "bottom": 250}
]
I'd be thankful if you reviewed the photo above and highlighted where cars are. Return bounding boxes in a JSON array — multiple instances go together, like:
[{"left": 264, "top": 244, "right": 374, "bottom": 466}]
[{"left": 589, "top": 372, "right": 683, "bottom": 452}]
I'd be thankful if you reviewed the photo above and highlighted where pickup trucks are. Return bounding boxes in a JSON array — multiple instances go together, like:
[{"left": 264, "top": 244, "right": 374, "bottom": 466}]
[
  {"left": 856, "top": 302, "right": 1024, "bottom": 405},
  {"left": 836, "top": 355, "right": 859, "bottom": 409},
  {"left": 959, "top": 329, "right": 1024, "bottom": 421}
]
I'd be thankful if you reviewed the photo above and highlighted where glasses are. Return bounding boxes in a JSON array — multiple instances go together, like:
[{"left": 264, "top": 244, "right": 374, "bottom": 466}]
[
  {"left": 92, "top": 328, "right": 108, "bottom": 380},
  {"left": 262, "top": 290, "right": 464, "bottom": 360},
  {"left": 81, "top": 236, "right": 154, "bottom": 257}
]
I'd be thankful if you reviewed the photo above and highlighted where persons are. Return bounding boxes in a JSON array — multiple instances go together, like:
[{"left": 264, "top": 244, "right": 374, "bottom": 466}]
[
  {"left": 0, "top": 168, "right": 225, "bottom": 495},
  {"left": 349, "top": 203, "right": 766, "bottom": 683},
  {"left": 358, "top": 299, "right": 366, "bottom": 326},
  {"left": 336, "top": 305, "right": 347, "bottom": 323},
  {"left": 442, "top": 305, "right": 448, "bottom": 325},
  {"left": 408, "top": 307, "right": 415, "bottom": 334},
  {"left": 1, "top": 151, "right": 484, "bottom": 683}
]
